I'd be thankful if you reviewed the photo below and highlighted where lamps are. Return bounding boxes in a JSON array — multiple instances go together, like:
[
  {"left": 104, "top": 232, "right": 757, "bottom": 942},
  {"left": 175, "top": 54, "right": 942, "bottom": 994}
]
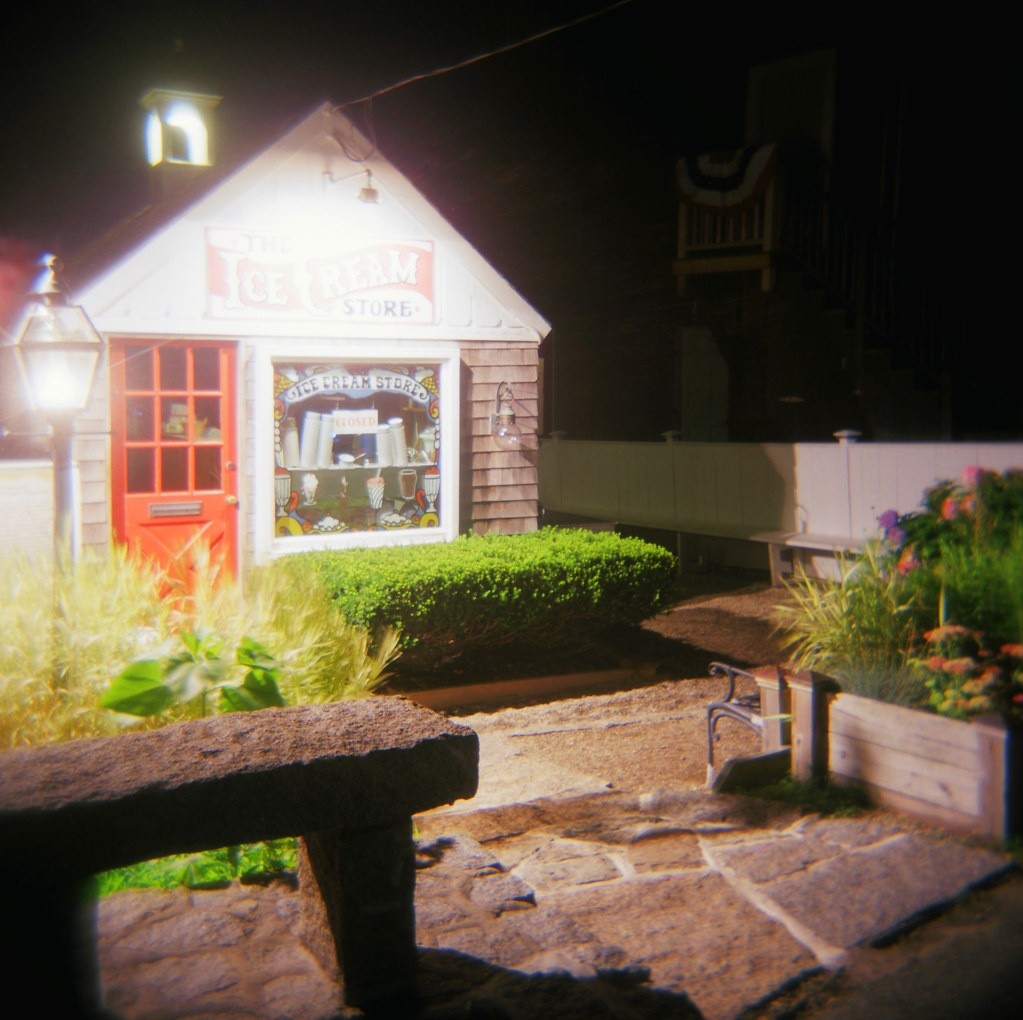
[
  {"left": 489, "top": 380, "right": 521, "bottom": 451},
  {"left": 330, "top": 168, "right": 380, "bottom": 205}
]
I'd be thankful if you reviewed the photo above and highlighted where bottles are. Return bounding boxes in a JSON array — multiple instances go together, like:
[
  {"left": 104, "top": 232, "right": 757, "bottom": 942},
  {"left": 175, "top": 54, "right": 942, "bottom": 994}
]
[
  {"left": 282, "top": 416, "right": 300, "bottom": 468},
  {"left": 377, "top": 417, "right": 409, "bottom": 467},
  {"left": 300, "top": 411, "right": 333, "bottom": 469}
]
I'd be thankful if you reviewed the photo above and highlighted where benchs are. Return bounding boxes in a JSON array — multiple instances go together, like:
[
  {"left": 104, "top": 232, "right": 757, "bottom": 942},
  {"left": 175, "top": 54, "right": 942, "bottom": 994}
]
[
  {"left": 705, "top": 662, "right": 763, "bottom": 782},
  {"left": 0, "top": 693, "right": 479, "bottom": 1020},
  {"left": 618, "top": 518, "right": 867, "bottom": 587}
]
